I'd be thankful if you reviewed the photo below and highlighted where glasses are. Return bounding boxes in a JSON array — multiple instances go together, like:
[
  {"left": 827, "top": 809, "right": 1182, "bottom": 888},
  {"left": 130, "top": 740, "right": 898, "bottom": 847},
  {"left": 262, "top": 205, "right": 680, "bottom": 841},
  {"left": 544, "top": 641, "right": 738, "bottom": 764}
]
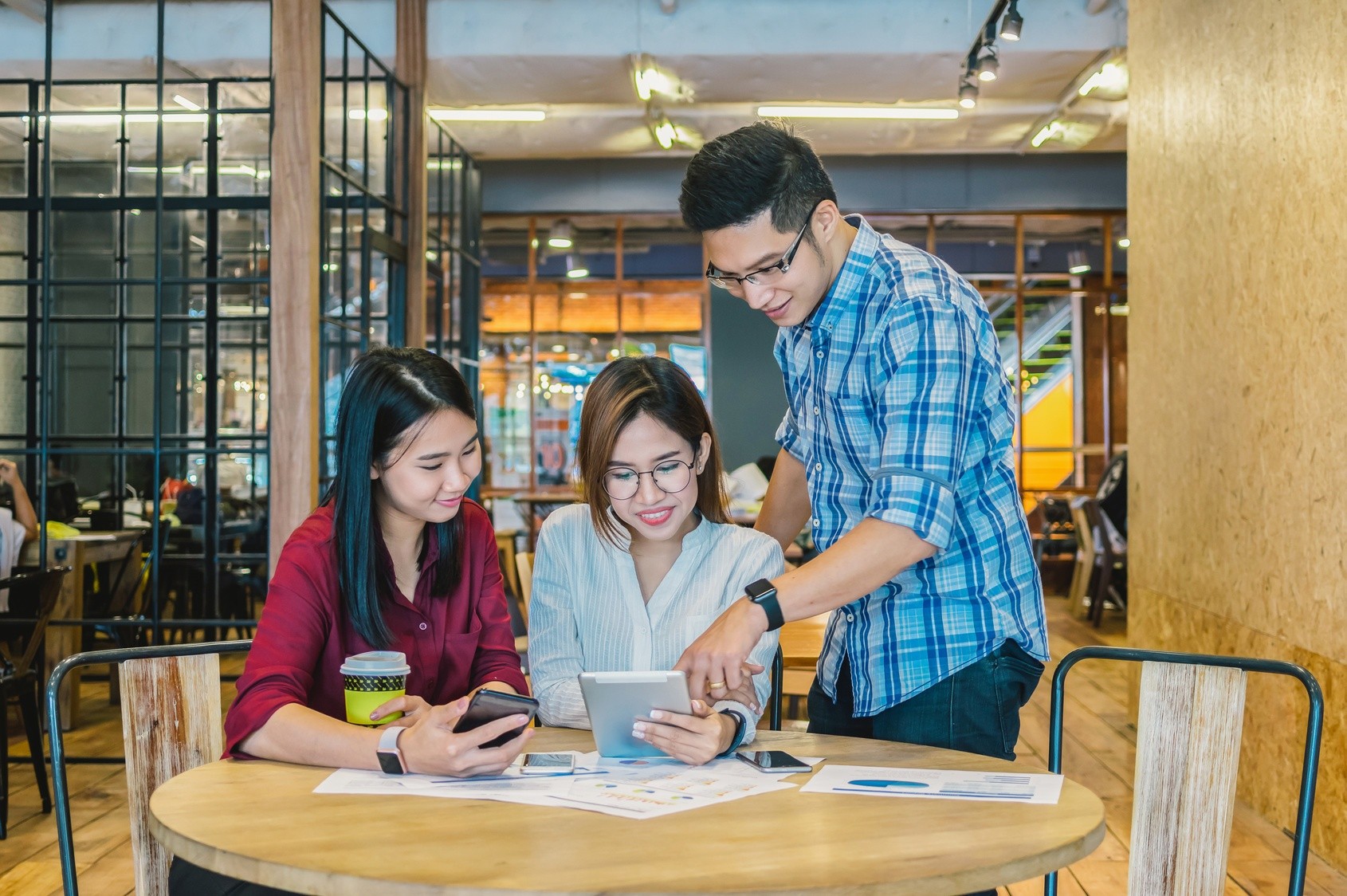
[
  {"left": 600, "top": 445, "right": 699, "bottom": 500},
  {"left": 704, "top": 199, "right": 826, "bottom": 290}
]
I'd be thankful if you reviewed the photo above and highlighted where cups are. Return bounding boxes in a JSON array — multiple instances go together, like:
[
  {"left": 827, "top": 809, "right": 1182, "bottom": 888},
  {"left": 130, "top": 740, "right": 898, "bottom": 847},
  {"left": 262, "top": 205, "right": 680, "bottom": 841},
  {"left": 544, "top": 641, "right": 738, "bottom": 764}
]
[{"left": 340, "top": 649, "right": 411, "bottom": 731}]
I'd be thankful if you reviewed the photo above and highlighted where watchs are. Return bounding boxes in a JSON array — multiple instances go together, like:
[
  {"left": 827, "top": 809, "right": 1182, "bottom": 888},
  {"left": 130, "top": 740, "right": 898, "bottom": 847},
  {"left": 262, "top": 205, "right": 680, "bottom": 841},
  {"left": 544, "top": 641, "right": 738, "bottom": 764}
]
[
  {"left": 375, "top": 726, "right": 409, "bottom": 777},
  {"left": 744, "top": 578, "right": 785, "bottom": 634}
]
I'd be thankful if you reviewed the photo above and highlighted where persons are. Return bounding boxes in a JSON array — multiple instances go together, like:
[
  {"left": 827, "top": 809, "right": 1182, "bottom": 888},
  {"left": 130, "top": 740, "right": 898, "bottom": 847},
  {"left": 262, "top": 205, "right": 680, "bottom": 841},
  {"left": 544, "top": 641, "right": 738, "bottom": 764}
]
[
  {"left": 0, "top": 458, "right": 40, "bottom": 614},
  {"left": 519, "top": 354, "right": 791, "bottom": 771},
  {"left": 37, "top": 443, "right": 83, "bottom": 524},
  {"left": 161, "top": 343, "right": 544, "bottom": 896},
  {"left": 668, "top": 113, "right": 1053, "bottom": 896}
]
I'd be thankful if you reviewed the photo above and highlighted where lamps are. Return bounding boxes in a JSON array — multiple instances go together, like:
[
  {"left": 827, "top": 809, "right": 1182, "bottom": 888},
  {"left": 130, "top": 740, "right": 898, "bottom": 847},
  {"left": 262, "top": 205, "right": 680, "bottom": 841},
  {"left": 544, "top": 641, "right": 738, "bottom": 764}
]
[{"left": 959, "top": 1, "right": 1023, "bottom": 107}]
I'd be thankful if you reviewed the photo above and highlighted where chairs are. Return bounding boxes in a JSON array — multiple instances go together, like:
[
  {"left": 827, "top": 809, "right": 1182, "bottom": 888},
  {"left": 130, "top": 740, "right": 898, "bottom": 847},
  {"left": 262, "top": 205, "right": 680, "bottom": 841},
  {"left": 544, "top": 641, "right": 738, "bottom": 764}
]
[
  {"left": 48, "top": 637, "right": 274, "bottom": 896},
  {"left": 1048, "top": 645, "right": 1325, "bottom": 895},
  {"left": 1063, "top": 495, "right": 1127, "bottom": 628},
  {"left": 2, "top": 502, "right": 248, "bottom": 843}
]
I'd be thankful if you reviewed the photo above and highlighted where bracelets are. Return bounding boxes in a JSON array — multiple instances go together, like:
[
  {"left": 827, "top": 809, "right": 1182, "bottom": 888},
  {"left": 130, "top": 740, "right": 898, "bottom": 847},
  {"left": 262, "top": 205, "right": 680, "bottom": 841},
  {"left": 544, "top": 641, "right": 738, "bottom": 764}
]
[{"left": 714, "top": 706, "right": 748, "bottom": 760}]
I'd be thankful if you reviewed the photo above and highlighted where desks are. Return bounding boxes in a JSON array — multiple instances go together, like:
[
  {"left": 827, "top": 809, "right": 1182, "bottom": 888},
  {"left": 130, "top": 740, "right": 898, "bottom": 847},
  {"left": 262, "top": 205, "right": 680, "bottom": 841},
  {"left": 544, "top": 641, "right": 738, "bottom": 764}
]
[{"left": 146, "top": 709, "right": 1108, "bottom": 895}]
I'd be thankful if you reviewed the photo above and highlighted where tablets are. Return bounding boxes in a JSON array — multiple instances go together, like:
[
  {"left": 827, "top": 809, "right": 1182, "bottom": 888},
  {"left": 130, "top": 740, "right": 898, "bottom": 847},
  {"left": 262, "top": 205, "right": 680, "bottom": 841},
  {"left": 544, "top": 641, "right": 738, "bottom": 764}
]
[{"left": 577, "top": 671, "right": 695, "bottom": 757}]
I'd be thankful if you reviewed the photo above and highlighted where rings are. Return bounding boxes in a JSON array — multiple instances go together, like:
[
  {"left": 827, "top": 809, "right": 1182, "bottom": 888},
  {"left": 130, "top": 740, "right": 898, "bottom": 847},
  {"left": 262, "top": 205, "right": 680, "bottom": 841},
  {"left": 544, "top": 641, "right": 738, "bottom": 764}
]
[{"left": 707, "top": 679, "right": 726, "bottom": 691}]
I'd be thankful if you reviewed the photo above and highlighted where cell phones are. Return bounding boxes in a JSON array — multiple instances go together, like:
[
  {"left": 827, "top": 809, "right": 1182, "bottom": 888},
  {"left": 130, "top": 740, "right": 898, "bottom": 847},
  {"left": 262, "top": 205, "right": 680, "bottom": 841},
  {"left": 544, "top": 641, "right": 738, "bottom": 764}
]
[
  {"left": 452, "top": 686, "right": 540, "bottom": 748},
  {"left": 736, "top": 751, "right": 812, "bottom": 774},
  {"left": 520, "top": 751, "right": 576, "bottom": 775}
]
